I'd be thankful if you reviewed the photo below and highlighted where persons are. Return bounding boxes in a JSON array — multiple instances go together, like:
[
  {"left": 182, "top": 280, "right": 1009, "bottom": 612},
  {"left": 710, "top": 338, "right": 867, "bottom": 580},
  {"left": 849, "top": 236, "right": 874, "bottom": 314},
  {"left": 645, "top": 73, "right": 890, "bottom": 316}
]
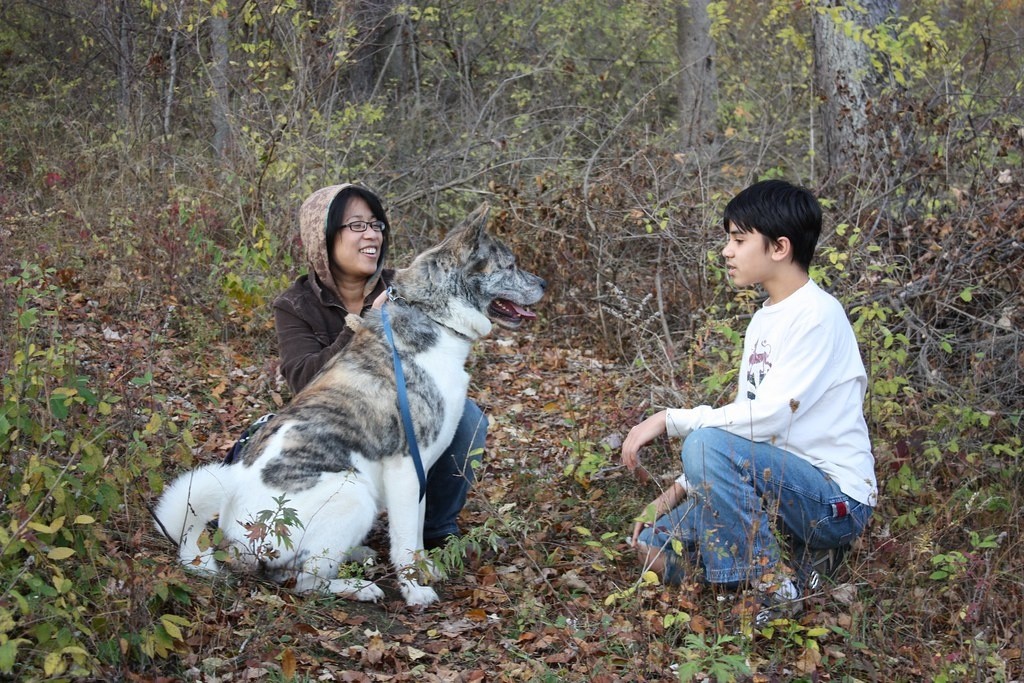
[
  {"left": 272, "top": 182, "right": 488, "bottom": 552},
  {"left": 620, "top": 180, "right": 877, "bottom": 634}
]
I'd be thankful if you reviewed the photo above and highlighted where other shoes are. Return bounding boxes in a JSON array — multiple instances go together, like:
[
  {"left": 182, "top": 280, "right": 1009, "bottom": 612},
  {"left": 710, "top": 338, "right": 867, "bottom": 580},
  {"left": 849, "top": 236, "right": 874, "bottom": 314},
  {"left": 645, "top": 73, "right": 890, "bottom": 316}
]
[{"left": 733, "top": 562, "right": 818, "bottom": 643}]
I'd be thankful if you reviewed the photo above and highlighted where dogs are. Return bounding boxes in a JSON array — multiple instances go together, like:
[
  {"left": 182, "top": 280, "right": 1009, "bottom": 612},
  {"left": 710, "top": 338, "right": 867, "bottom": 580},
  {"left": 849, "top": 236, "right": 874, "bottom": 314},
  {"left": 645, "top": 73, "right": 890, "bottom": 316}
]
[{"left": 152, "top": 200, "right": 545, "bottom": 605}]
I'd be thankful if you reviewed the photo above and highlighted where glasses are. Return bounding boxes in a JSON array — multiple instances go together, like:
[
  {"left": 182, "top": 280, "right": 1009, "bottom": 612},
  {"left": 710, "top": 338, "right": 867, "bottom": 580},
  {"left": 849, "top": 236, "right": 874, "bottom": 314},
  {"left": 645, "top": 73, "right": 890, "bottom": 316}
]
[{"left": 338, "top": 220, "right": 387, "bottom": 232}]
